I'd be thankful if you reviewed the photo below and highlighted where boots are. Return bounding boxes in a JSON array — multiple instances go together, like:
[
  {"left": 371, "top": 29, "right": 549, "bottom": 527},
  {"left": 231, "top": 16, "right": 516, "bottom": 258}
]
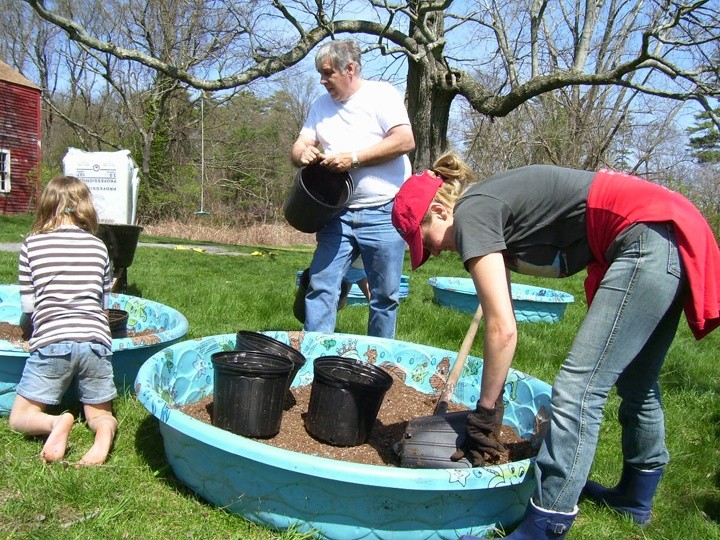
[
  {"left": 585, "top": 463, "right": 661, "bottom": 524},
  {"left": 460, "top": 497, "right": 578, "bottom": 540}
]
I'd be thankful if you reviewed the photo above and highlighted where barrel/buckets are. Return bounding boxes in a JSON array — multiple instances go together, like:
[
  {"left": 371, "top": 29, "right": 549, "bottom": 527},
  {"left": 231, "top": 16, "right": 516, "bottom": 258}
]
[
  {"left": 292, "top": 269, "right": 355, "bottom": 324},
  {"left": 232, "top": 329, "right": 307, "bottom": 403},
  {"left": 211, "top": 350, "right": 295, "bottom": 439},
  {"left": 101, "top": 306, "right": 128, "bottom": 339},
  {"left": 19, "top": 311, "right": 36, "bottom": 341},
  {"left": 399, "top": 409, "right": 484, "bottom": 470},
  {"left": 283, "top": 156, "right": 354, "bottom": 233},
  {"left": 304, "top": 355, "right": 393, "bottom": 447}
]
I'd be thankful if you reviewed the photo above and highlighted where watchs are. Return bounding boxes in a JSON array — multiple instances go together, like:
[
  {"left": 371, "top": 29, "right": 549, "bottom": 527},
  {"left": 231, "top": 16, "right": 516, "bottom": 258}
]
[{"left": 351, "top": 154, "right": 360, "bottom": 168}]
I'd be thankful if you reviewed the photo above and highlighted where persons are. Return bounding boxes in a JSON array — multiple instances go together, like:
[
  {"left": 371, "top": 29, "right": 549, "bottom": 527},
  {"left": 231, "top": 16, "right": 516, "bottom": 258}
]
[
  {"left": 291, "top": 234, "right": 370, "bottom": 323},
  {"left": 7, "top": 175, "right": 118, "bottom": 469},
  {"left": 291, "top": 37, "right": 417, "bottom": 339},
  {"left": 391, "top": 154, "right": 712, "bottom": 540}
]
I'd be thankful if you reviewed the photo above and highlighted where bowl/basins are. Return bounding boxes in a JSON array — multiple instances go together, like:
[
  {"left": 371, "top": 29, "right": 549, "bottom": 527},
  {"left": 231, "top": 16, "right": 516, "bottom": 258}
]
[
  {"left": 134, "top": 329, "right": 566, "bottom": 540},
  {"left": 428, "top": 275, "right": 575, "bottom": 325},
  {"left": 0, "top": 283, "right": 189, "bottom": 417},
  {"left": 294, "top": 268, "right": 412, "bottom": 308}
]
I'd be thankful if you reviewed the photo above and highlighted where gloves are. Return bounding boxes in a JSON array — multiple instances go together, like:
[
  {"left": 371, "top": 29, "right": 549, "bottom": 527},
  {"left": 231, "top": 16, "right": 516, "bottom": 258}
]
[{"left": 452, "top": 400, "right": 504, "bottom": 466}]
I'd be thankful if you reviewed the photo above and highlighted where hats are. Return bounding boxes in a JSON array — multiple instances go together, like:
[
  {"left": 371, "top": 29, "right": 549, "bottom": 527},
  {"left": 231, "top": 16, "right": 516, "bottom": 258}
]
[{"left": 391, "top": 170, "right": 444, "bottom": 271}]
